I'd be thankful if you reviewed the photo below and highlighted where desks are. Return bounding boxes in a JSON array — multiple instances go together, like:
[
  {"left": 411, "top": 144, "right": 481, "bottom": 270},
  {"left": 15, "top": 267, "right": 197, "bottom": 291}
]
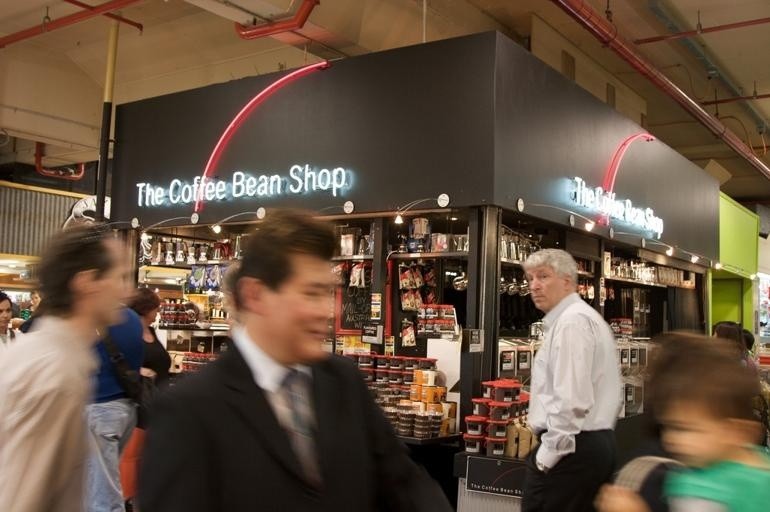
[{"left": 395, "top": 429, "right": 465, "bottom": 511}]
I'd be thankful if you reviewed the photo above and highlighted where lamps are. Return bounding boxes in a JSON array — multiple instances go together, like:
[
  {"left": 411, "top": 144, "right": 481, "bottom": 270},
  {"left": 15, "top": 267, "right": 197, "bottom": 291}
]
[
  {"left": 682, "top": 247, "right": 722, "bottom": 270},
  {"left": 398, "top": 193, "right": 450, "bottom": 216},
  {"left": 216, "top": 206, "right": 266, "bottom": 226},
  {"left": 315, "top": 201, "right": 355, "bottom": 215},
  {"left": 145, "top": 211, "right": 199, "bottom": 226},
  {"left": 529, "top": 202, "right": 588, "bottom": 231},
  {"left": 646, "top": 240, "right": 698, "bottom": 264},
  {"left": 614, "top": 230, "right": 674, "bottom": 256},
  {"left": 105, "top": 217, "right": 141, "bottom": 229}
]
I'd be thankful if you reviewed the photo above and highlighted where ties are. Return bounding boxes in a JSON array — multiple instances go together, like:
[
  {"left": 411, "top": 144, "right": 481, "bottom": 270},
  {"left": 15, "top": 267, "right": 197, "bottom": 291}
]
[{"left": 271, "top": 368, "right": 331, "bottom": 495}]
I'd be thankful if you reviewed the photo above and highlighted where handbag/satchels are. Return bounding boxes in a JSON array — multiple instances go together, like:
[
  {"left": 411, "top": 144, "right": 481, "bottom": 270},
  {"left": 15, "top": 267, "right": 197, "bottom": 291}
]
[{"left": 120, "top": 369, "right": 166, "bottom": 430}]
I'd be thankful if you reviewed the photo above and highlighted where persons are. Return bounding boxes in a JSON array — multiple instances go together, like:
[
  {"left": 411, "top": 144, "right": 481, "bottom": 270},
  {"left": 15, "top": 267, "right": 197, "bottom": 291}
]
[
  {"left": 0, "top": 223, "right": 174, "bottom": 512},
  {"left": 135, "top": 201, "right": 453, "bottom": 511},
  {"left": 592, "top": 325, "right": 769, "bottom": 512},
  {"left": 705, "top": 316, "right": 752, "bottom": 351},
  {"left": 518, "top": 247, "right": 623, "bottom": 511}
]
